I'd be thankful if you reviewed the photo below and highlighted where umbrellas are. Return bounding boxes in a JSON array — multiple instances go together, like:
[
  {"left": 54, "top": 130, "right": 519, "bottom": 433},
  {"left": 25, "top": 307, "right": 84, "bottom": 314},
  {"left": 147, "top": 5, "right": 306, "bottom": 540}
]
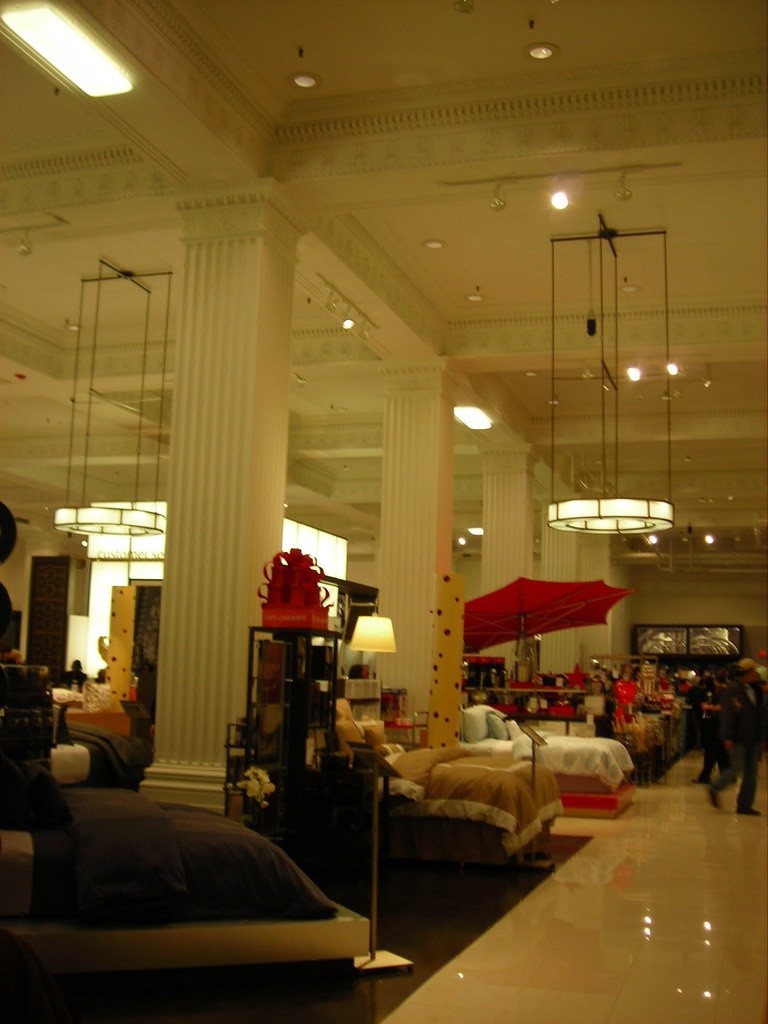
[{"left": 464, "top": 578, "right": 637, "bottom": 707}]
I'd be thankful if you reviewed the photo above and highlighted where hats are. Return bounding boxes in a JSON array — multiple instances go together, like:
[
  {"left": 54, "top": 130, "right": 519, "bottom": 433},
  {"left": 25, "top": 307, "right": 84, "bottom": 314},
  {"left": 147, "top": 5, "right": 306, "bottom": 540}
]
[{"left": 736, "top": 658, "right": 761, "bottom": 671}]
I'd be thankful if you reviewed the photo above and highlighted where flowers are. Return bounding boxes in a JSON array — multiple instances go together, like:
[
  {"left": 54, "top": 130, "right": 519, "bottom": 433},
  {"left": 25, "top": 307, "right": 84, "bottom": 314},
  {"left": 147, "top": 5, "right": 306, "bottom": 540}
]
[{"left": 227, "top": 763, "right": 277, "bottom": 809}]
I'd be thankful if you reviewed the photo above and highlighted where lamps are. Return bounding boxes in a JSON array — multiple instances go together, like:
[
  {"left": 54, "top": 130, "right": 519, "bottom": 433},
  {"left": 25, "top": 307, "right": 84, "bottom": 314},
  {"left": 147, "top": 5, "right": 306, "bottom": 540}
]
[
  {"left": 547, "top": 212, "right": 675, "bottom": 536},
  {"left": 15, "top": 229, "right": 36, "bottom": 256},
  {"left": 486, "top": 178, "right": 516, "bottom": 213},
  {"left": 612, "top": 168, "right": 633, "bottom": 203},
  {"left": 53, "top": 257, "right": 172, "bottom": 538},
  {"left": 349, "top": 614, "right": 397, "bottom": 680}
]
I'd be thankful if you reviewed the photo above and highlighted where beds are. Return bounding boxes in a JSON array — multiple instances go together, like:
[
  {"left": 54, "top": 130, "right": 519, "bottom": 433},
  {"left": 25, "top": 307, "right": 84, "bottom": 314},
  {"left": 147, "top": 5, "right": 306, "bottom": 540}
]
[
  {"left": 0, "top": 753, "right": 371, "bottom": 993},
  {"left": 460, "top": 714, "right": 637, "bottom": 794},
  {"left": 315, "top": 718, "right": 563, "bottom": 868}
]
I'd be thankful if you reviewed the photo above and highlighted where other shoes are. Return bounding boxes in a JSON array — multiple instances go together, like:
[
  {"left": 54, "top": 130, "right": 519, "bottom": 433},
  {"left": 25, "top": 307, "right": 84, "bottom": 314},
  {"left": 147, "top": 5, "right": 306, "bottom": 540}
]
[
  {"left": 708, "top": 787, "right": 721, "bottom": 810},
  {"left": 736, "top": 806, "right": 761, "bottom": 816},
  {"left": 692, "top": 777, "right": 711, "bottom": 785}
]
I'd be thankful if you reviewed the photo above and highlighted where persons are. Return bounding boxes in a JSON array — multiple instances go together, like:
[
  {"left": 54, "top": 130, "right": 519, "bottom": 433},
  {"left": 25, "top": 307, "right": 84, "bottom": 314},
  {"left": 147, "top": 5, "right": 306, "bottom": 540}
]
[
  {"left": 708, "top": 658, "right": 763, "bottom": 816},
  {"left": 677, "top": 669, "right": 768, "bottom": 783},
  {"left": 63, "top": 660, "right": 86, "bottom": 689}
]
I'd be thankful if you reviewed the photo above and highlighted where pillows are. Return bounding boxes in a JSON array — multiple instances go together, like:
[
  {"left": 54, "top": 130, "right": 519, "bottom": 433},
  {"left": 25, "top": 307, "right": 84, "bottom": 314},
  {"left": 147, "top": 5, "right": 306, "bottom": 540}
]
[
  {"left": 462, "top": 703, "right": 519, "bottom": 742},
  {"left": 361, "top": 723, "right": 387, "bottom": 746},
  {"left": 0, "top": 753, "right": 73, "bottom": 831}
]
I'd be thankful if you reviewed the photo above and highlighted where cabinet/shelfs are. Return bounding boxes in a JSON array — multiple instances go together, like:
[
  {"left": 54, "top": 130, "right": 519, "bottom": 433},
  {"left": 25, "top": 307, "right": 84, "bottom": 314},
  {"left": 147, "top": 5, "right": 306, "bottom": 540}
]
[{"left": 224, "top": 624, "right": 342, "bottom": 882}]
